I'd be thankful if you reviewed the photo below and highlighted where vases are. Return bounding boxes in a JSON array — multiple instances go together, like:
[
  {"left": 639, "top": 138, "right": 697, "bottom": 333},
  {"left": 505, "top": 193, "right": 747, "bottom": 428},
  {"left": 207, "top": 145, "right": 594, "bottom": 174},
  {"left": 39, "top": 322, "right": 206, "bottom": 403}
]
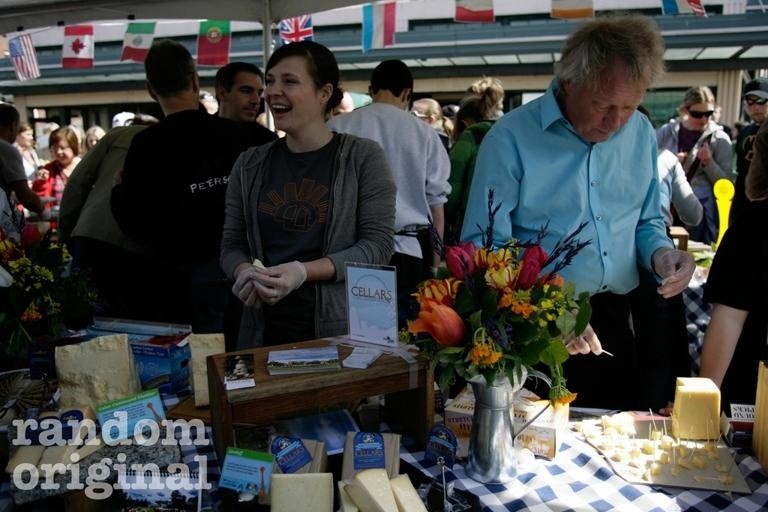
[{"left": 463, "top": 371, "right": 516, "bottom": 487}]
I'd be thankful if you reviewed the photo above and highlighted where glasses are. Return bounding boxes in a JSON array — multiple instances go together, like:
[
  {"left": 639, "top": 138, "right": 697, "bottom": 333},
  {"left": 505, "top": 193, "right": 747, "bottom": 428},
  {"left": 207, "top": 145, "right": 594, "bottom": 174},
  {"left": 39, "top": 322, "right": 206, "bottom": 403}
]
[
  {"left": 686, "top": 108, "right": 715, "bottom": 119},
  {"left": 742, "top": 96, "right": 768, "bottom": 106}
]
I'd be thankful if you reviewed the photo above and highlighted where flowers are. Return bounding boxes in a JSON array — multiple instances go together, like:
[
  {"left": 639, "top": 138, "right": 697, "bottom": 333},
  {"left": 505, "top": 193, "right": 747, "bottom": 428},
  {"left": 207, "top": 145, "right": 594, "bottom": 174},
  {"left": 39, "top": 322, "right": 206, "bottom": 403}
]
[
  {"left": 389, "top": 187, "right": 594, "bottom": 410},
  {"left": 0, "top": 222, "right": 95, "bottom": 324}
]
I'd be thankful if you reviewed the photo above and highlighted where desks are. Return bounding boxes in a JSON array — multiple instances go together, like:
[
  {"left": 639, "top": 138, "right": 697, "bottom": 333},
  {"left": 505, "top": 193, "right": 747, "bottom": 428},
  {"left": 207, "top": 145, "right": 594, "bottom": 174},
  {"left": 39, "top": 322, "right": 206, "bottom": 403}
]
[
  {"left": 0, "top": 371, "right": 766, "bottom": 512},
  {"left": 669, "top": 234, "right": 715, "bottom": 379}
]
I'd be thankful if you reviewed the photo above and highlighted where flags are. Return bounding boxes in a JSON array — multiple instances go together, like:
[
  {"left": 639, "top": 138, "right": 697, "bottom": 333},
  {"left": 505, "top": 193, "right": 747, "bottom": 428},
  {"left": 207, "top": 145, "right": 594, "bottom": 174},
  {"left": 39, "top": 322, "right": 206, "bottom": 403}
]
[
  {"left": 453, "top": 0, "right": 496, "bottom": 25},
  {"left": 362, "top": 0, "right": 398, "bottom": 54},
  {"left": 659, "top": 1, "right": 708, "bottom": 19},
  {"left": 551, "top": 1, "right": 594, "bottom": 20}
]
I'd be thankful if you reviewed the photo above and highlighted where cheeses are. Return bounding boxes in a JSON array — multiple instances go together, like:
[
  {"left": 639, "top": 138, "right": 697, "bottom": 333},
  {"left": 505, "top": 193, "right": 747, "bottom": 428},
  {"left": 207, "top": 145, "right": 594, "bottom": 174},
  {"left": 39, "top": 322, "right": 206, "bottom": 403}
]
[
  {"left": 574, "top": 375, "right": 733, "bottom": 485},
  {"left": 259, "top": 431, "right": 429, "bottom": 512},
  {"left": 188, "top": 332, "right": 226, "bottom": 407},
  {"left": 5, "top": 334, "right": 142, "bottom": 476}
]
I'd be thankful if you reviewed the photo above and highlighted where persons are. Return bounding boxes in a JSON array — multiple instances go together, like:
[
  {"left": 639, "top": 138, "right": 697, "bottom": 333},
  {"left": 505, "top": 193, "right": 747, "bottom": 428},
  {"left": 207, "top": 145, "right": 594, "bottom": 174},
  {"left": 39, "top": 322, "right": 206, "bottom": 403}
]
[{"left": 0, "top": 13, "right": 768, "bottom": 416}]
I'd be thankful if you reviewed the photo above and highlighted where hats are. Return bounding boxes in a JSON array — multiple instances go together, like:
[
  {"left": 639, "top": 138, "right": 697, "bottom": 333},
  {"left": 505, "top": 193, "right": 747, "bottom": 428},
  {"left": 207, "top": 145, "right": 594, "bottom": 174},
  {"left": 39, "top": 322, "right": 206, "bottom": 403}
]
[{"left": 741, "top": 76, "right": 768, "bottom": 96}]
